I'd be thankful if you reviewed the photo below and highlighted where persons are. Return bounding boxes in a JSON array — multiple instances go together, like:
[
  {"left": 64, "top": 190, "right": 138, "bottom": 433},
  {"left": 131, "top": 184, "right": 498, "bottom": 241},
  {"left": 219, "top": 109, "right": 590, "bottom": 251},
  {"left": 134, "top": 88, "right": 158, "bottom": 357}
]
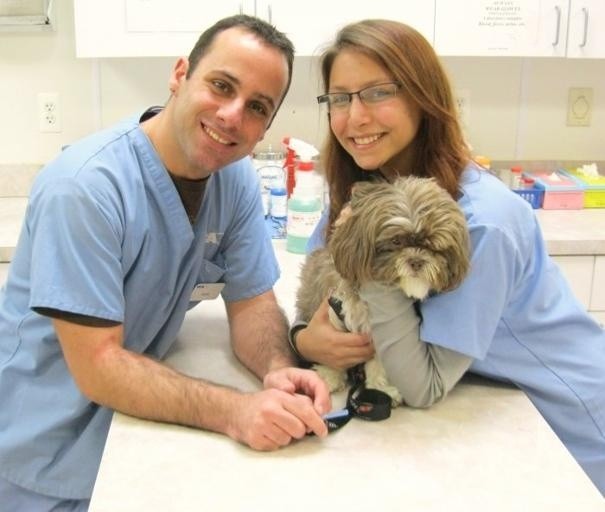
[
  {"left": 286, "top": 19, "right": 604, "bottom": 497},
  {"left": 1, "top": 15, "right": 333, "bottom": 512}
]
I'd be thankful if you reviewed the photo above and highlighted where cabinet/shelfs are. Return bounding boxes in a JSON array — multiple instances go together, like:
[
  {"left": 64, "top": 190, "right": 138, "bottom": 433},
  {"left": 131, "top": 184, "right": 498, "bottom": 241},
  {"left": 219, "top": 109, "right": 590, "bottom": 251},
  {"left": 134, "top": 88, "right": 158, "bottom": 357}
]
[
  {"left": 549, "top": 256, "right": 604, "bottom": 331},
  {"left": 71, "top": 1, "right": 434, "bottom": 61},
  {"left": 435, "top": 1, "right": 605, "bottom": 62}
]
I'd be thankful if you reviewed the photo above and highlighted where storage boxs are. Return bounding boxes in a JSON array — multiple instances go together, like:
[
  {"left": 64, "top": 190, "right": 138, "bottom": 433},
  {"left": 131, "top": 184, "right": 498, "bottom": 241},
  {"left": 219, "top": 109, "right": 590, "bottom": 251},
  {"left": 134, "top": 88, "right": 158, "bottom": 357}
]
[{"left": 524, "top": 169, "right": 605, "bottom": 208}]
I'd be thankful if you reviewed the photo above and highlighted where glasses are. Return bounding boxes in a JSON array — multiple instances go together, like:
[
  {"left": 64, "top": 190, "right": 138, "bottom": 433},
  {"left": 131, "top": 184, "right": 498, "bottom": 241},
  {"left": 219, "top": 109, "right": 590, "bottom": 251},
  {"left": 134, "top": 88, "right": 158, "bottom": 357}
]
[{"left": 317, "top": 81, "right": 402, "bottom": 112}]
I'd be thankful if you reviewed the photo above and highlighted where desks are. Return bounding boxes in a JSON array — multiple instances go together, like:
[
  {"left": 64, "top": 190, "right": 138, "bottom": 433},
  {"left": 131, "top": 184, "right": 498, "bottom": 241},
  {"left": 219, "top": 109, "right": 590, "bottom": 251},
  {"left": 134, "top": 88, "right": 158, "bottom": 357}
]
[{"left": 88, "top": 291, "right": 605, "bottom": 510}]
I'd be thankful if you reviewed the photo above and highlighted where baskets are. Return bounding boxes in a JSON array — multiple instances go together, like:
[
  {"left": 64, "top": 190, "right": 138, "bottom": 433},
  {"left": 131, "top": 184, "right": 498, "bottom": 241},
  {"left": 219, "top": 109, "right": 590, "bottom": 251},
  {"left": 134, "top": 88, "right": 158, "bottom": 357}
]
[{"left": 514, "top": 183, "right": 545, "bottom": 209}]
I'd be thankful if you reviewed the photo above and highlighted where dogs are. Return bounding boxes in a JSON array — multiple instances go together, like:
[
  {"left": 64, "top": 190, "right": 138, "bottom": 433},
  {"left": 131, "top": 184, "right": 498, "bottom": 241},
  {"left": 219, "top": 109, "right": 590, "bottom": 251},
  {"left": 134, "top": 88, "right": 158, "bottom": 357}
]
[{"left": 293, "top": 170, "right": 471, "bottom": 410}]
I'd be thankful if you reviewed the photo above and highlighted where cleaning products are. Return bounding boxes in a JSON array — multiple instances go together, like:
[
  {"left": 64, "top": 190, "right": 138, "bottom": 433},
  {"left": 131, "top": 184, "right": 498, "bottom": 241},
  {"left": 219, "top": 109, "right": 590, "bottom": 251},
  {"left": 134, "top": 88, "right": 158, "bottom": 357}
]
[{"left": 281, "top": 137, "right": 323, "bottom": 253}]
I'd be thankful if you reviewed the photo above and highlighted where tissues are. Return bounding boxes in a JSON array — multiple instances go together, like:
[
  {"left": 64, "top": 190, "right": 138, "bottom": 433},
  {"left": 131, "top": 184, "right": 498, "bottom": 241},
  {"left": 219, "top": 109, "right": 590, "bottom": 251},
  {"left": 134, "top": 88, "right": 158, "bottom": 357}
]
[{"left": 567, "top": 163, "right": 605, "bottom": 208}]
